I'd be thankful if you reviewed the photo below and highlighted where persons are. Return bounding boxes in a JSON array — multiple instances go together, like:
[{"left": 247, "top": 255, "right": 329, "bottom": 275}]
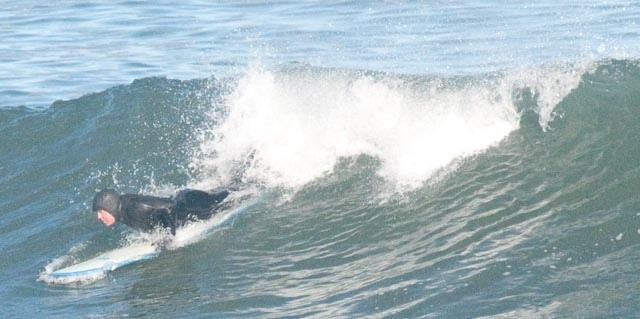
[{"left": 93, "top": 186, "right": 246, "bottom": 236}]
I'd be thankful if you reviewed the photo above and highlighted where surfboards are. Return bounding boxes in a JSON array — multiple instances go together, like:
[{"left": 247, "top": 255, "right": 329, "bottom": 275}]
[{"left": 48, "top": 198, "right": 258, "bottom": 284}]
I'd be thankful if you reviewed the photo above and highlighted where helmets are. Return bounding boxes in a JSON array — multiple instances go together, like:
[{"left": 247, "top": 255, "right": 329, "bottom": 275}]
[{"left": 93, "top": 189, "right": 120, "bottom": 221}]
